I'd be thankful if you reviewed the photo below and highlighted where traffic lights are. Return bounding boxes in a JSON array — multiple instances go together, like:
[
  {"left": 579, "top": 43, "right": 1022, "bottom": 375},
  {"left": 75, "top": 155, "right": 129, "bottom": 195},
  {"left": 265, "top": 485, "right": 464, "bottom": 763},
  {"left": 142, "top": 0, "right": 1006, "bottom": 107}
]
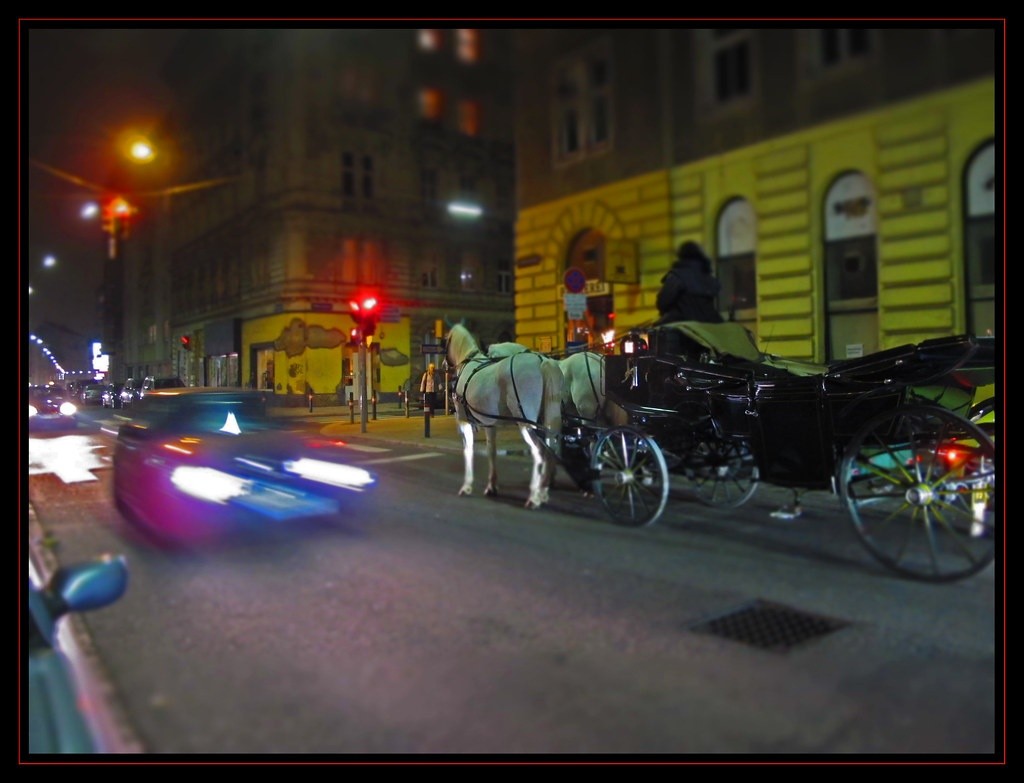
[
  {"left": 350, "top": 328, "right": 362, "bottom": 344},
  {"left": 181, "top": 336, "right": 190, "bottom": 350},
  {"left": 350, "top": 291, "right": 377, "bottom": 316}
]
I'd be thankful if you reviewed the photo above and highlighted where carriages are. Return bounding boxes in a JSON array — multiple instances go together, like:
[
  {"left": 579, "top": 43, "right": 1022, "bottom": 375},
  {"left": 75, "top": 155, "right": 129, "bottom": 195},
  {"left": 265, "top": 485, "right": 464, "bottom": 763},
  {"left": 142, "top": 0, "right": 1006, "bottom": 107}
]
[{"left": 440, "top": 314, "right": 998, "bottom": 586}]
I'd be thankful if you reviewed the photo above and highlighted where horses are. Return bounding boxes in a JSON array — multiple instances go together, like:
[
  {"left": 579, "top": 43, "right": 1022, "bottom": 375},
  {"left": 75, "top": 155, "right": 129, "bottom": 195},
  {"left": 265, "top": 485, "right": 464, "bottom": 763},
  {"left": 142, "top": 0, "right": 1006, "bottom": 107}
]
[{"left": 443, "top": 314, "right": 606, "bottom": 509}]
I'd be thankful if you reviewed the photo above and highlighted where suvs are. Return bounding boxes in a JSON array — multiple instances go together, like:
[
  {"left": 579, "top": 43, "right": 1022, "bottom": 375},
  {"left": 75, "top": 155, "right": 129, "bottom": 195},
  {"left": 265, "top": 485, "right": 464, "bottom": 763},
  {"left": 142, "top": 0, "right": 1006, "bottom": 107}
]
[
  {"left": 120, "top": 378, "right": 144, "bottom": 411},
  {"left": 73, "top": 378, "right": 100, "bottom": 399},
  {"left": 141, "top": 375, "right": 188, "bottom": 412}
]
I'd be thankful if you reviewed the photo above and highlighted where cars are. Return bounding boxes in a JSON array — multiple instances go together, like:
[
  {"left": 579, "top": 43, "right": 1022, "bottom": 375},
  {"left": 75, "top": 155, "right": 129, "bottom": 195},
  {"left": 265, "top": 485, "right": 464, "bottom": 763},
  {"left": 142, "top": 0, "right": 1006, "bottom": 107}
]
[
  {"left": 29, "top": 385, "right": 79, "bottom": 432},
  {"left": 101, "top": 383, "right": 122, "bottom": 409},
  {"left": 80, "top": 385, "right": 105, "bottom": 406},
  {"left": 113, "top": 386, "right": 378, "bottom": 547}
]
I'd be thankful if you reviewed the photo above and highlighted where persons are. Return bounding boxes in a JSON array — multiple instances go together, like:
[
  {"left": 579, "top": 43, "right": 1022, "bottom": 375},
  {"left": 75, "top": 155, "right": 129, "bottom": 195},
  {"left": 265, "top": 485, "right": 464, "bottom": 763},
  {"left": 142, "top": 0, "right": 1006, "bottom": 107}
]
[
  {"left": 653, "top": 240, "right": 722, "bottom": 324},
  {"left": 419, "top": 363, "right": 439, "bottom": 418}
]
